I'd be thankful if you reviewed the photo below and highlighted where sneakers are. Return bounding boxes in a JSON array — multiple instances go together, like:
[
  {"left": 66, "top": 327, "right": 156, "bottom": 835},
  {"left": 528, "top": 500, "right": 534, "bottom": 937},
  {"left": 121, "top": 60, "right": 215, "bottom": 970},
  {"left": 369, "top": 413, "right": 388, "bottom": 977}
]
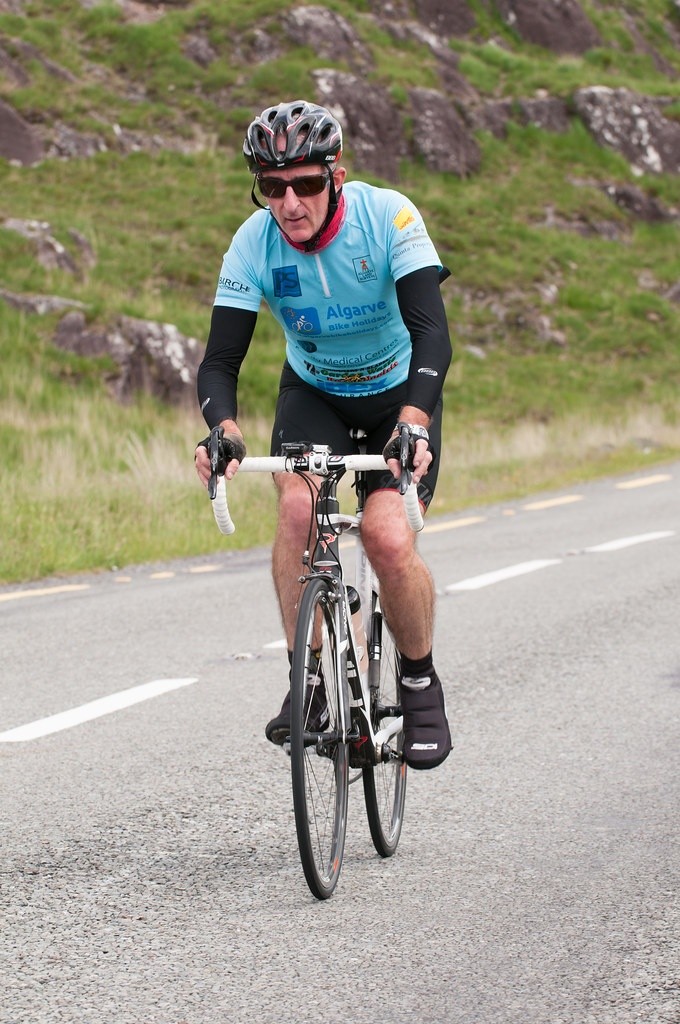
[
  {"left": 398, "top": 666, "right": 453, "bottom": 771},
  {"left": 265, "top": 670, "right": 331, "bottom": 746}
]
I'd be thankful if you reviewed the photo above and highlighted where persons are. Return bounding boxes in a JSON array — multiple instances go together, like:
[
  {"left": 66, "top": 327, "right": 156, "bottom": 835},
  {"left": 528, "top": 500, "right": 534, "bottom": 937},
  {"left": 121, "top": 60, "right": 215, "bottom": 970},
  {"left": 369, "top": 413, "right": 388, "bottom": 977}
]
[{"left": 193, "top": 101, "right": 452, "bottom": 770}]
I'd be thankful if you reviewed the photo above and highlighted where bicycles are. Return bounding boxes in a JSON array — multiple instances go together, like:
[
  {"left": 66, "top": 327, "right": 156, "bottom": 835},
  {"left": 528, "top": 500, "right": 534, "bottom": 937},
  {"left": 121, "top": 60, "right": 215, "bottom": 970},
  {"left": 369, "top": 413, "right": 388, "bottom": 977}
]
[{"left": 208, "top": 422, "right": 433, "bottom": 902}]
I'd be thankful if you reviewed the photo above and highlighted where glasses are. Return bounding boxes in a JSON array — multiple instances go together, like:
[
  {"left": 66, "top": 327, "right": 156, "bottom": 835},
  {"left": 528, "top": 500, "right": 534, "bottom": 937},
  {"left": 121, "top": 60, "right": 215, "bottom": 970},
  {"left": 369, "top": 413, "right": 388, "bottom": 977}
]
[{"left": 254, "top": 170, "right": 332, "bottom": 199}]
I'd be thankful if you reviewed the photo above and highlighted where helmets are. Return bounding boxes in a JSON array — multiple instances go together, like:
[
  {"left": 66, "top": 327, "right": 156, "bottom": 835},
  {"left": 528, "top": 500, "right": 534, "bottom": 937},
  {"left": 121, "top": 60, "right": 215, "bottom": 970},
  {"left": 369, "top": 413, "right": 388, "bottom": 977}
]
[{"left": 241, "top": 99, "right": 345, "bottom": 174}]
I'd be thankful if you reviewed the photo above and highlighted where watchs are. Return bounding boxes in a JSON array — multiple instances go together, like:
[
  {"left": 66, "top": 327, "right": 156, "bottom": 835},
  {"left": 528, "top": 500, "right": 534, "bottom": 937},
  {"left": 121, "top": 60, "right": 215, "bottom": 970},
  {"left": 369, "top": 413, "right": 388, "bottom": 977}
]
[{"left": 395, "top": 423, "right": 429, "bottom": 440}]
